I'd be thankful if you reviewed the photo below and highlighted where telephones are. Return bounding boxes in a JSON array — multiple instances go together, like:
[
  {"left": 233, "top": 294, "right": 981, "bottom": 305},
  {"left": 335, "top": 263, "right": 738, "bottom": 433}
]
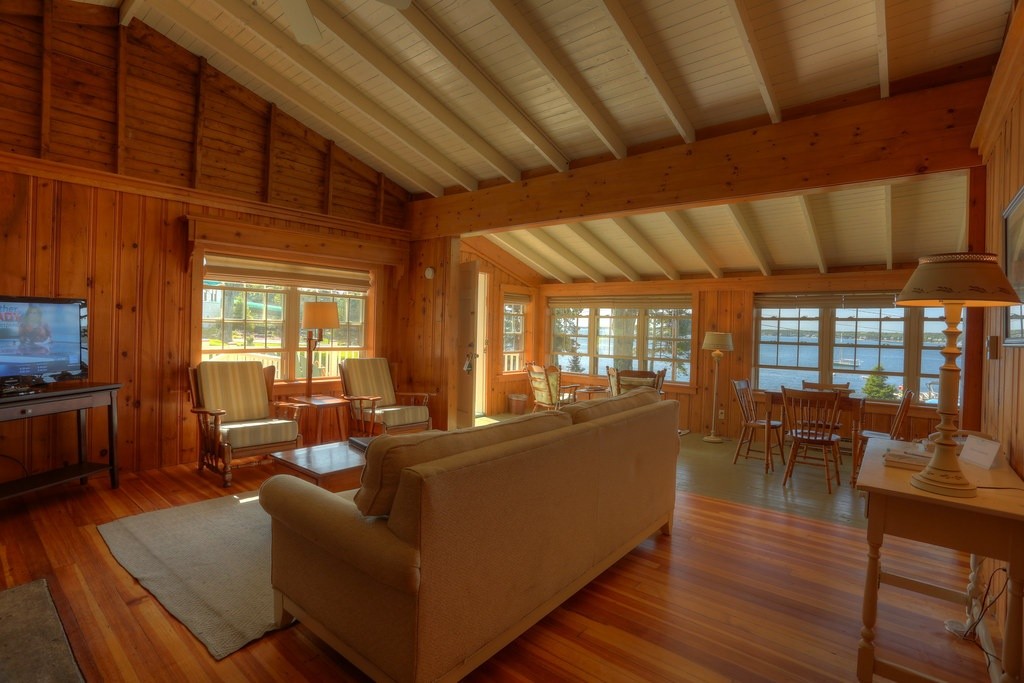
[{"left": 922, "top": 430, "right": 996, "bottom": 454}]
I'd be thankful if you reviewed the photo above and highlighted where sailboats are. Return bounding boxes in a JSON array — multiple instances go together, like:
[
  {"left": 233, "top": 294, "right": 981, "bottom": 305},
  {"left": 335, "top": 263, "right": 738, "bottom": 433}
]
[{"left": 837, "top": 333, "right": 861, "bottom": 367}]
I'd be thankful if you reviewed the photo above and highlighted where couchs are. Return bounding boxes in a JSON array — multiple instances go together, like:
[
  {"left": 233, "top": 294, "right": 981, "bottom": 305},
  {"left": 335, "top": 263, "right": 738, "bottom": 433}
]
[{"left": 258, "top": 386, "right": 681, "bottom": 683}]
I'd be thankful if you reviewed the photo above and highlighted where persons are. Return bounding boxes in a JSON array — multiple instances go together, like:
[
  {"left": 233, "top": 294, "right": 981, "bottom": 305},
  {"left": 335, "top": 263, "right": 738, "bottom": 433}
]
[{"left": 18, "top": 306, "right": 51, "bottom": 355}]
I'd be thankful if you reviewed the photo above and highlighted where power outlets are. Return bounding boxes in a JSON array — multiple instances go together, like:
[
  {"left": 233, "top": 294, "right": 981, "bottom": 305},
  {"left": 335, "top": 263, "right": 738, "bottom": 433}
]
[{"left": 719, "top": 409, "right": 725, "bottom": 419}]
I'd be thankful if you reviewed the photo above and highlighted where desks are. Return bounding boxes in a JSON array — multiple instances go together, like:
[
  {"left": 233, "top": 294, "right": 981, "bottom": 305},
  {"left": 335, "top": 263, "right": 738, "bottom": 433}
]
[
  {"left": 856, "top": 437, "right": 1024, "bottom": 683},
  {"left": 574, "top": 388, "right": 665, "bottom": 400},
  {"left": 0, "top": 382, "right": 123, "bottom": 501},
  {"left": 764, "top": 387, "right": 868, "bottom": 474},
  {"left": 289, "top": 395, "right": 350, "bottom": 445}
]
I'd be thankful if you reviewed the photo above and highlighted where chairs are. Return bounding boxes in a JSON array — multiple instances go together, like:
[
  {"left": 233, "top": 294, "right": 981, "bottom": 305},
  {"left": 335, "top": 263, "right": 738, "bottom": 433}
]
[
  {"left": 525, "top": 363, "right": 667, "bottom": 414},
  {"left": 338, "top": 357, "right": 434, "bottom": 439},
  {"left": 185, "top": 361, "right": 305, "bottom": 490},
  {"left": 730, "top": 378, "right": 915, "bottom": 494}
]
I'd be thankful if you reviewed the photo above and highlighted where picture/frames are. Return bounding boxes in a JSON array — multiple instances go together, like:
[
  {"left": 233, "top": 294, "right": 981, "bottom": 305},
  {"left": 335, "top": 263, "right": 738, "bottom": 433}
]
[{"left": 1000, "top": 182, "right": 1024, "bottom": 347}]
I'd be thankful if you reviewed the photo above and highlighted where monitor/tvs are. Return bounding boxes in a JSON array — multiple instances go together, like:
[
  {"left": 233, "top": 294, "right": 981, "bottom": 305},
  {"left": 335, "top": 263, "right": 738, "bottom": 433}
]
[{"left": 0, "top": 295, "right": 88, "bottom": 386}]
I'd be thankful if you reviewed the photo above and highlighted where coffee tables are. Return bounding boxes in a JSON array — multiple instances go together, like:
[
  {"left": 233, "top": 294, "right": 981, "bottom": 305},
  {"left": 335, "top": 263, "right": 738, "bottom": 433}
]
[{"left": 269, "top": 441, "right": 367, "bottom": 486}]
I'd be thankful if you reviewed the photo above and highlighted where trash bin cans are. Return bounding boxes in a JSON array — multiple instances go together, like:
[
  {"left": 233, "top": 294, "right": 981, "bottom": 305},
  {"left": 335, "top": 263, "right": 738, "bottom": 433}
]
[{"left": 508, "top": 394, "right": 527, "bottom": 415}]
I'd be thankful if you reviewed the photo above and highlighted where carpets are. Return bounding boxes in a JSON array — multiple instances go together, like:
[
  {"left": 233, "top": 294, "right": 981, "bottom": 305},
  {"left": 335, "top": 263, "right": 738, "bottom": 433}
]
[
  {"left": 97, "top": 489, "right": 299, "bottom": 662},
  {"left": 0, "top": 577, "right": 86, "bottom": 683}
]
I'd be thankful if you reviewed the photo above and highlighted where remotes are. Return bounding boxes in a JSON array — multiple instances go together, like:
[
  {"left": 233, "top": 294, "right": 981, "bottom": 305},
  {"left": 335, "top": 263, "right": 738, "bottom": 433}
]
[{"left": 0, "top": 387, "right": 31, "bottom": 394}]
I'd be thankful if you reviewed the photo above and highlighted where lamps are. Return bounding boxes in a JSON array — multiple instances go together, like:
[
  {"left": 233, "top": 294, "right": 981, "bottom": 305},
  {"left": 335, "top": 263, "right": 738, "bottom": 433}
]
[
  {"left": 702, "top": 332, "right": 734, "bottom": 444},
  {"left": 894, "top": 250, "right": 1024, "bottom": 500},
  {"left": 302, "top": 301, "right": 341, "bottom": 396}
]
